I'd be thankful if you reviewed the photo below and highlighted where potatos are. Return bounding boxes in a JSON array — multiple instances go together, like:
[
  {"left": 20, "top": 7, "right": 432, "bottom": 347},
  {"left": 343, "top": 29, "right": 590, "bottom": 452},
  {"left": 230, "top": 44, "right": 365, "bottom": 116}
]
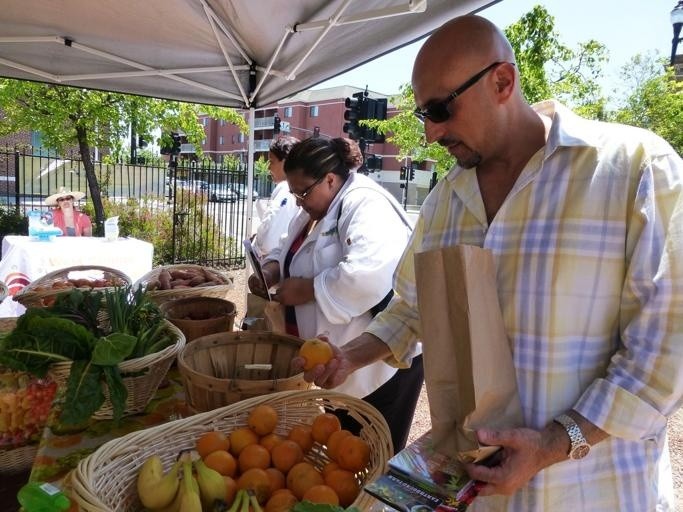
[{"left": 145, "top": 266, "right": 228, "bottom": 292}]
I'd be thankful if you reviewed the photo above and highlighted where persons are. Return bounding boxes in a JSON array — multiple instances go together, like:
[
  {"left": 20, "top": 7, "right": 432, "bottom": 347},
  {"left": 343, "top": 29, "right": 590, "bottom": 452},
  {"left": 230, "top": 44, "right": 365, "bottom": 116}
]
[
  {"left": 289, "top": 12, "right": 682, "bottom": 512},
  {"left": 251, "top": 136, "right": 304, "bottom": 265},
  {"left": 43, "top": 186, "right": 93, "bottom": 237},
  {"left": 245, "top": 136, "right": 426, "bottom": 473}
]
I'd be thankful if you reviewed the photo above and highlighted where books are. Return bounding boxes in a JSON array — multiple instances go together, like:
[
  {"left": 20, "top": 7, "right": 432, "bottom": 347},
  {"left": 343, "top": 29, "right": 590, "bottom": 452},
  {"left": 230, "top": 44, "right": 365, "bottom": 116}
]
[
  {"left": 242, "top": 240, "right": 272, "bottom": 303},
  {"left": 385, "top": 426, "right": 502, "bottom": 501},
  {"left": 358, "top": 468, "right": 488, "bottom": 512}
]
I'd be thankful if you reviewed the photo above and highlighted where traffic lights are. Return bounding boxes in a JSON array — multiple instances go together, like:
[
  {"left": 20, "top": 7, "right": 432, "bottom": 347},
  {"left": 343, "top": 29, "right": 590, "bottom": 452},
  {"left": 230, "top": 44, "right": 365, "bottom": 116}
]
[
  {"left": 138, "top": 134, "right": 147, "bottom": 147},
  {"left": 409, "top": 167, "right": 414, "bottom": 180},
  {"left": 273, "top": 115, "right": 281, "bottom": 135},
  {"left": 399, "top": 166, "right": 405, "bottom": 181},
  {"left": 171, "top": 133, "right": 181, "bottom": 155},
  {"left": 368, "top": 155, "right": 382, "bottom": 172},
  {"left": 314, "top": 126, "right": 320, "bottom": 138},
  {"left": 341, "top": 91, "right": 366, "bottom": 139}
]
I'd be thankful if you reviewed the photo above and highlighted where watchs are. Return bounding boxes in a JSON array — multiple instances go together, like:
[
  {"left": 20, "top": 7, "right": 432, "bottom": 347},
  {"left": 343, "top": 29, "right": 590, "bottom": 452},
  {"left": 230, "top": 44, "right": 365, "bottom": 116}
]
[{"left": 549, "top": 413, "right": 591, "bottom": 463}]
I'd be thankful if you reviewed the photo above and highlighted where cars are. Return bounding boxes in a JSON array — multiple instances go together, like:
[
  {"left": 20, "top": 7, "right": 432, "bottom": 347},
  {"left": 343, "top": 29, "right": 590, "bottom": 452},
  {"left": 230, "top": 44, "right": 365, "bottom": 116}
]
[{"left": 164, "top": 176, "right": 259, "bottom": 205}]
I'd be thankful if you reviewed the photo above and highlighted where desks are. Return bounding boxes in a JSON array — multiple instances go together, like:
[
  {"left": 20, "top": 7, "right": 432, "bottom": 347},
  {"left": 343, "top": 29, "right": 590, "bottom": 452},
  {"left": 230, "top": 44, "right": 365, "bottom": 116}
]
[
  {"left": 16, "top": 368, "right": 316, "bottom": 512},
  {"left": 0, "top": 233, "right": 154, "bottom": 319}
]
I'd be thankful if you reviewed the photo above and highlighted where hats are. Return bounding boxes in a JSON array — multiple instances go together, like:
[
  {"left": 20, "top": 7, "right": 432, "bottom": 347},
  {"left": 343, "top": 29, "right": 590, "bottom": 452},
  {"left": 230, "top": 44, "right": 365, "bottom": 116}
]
[{"left": 44, "top": 186, "right": 86, "bottom": 205}]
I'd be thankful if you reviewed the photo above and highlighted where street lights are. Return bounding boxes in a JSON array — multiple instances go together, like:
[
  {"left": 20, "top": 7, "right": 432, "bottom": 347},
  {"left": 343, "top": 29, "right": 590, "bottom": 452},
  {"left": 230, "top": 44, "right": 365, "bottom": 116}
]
[{"left": 666, "top": 0, "right": 683, "bottom": 67}]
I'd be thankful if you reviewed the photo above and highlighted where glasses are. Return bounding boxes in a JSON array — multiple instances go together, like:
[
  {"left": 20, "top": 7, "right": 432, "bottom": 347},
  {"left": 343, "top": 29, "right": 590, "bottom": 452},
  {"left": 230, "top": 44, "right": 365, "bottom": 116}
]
[
  {"left": 288, "top": 171, "right": 337, "bottom": 202},
  {"left": 57, "top": 196, "right": 72, "bottom": 202},
  {"left": 412, "top": 61, "right": 515, "bottom": 125}
]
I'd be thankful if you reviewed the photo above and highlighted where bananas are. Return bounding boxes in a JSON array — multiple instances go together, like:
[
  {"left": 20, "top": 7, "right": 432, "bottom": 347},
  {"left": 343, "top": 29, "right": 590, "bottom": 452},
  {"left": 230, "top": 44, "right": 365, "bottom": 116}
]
[{"left": 138, "top": 448, "right": 227, "bottom": 512}]
